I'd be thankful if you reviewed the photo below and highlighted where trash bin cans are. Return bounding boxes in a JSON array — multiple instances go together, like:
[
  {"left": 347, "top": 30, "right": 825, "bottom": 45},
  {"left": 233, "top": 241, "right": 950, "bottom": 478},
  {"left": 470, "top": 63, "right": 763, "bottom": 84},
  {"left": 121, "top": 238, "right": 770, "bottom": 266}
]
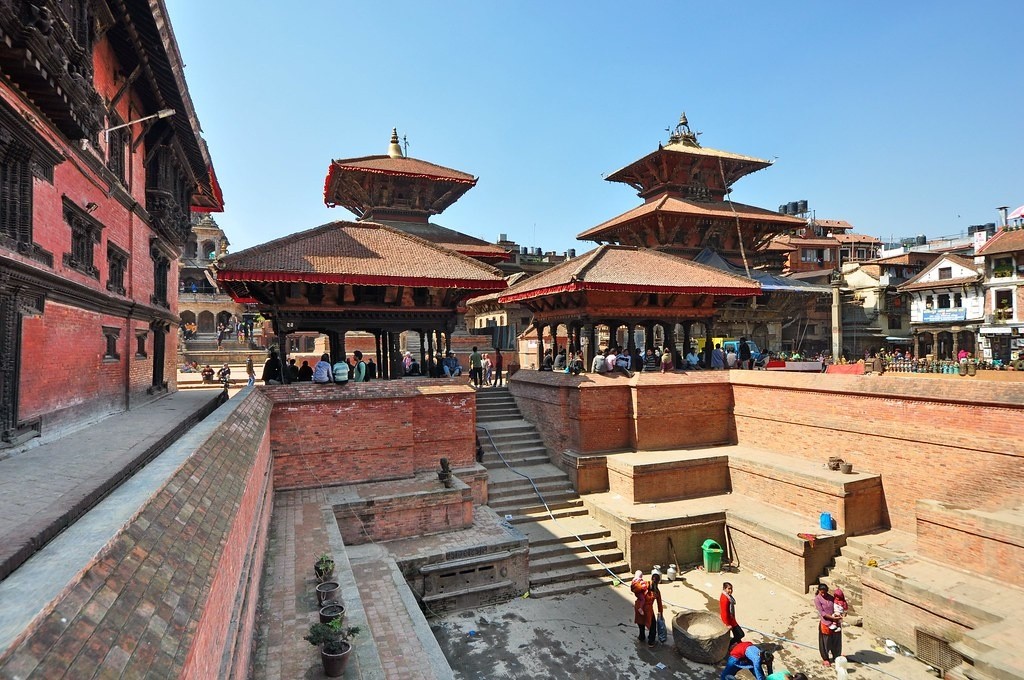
[
  {"left": 926, "top": 354, "right": 934, "bottom": 362},
  {"left": 701, "top": 539, "right": 724, "bottom": 572}
]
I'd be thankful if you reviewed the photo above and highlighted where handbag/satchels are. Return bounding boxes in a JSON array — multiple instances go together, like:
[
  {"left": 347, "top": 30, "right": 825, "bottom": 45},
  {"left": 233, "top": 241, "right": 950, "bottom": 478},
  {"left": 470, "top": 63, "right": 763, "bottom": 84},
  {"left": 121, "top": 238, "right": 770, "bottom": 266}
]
[
  {"left": 657, "top": 612, "right": 667, "bottom": 643},
  {"left": 819, "top": 513, "right": 833, "bottom": 530}
]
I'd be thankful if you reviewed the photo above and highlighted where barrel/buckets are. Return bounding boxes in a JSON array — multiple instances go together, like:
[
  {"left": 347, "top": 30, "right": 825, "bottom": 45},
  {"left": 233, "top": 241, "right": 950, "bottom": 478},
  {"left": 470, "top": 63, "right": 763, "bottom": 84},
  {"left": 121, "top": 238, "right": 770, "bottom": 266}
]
[
  {"left": 667, "top": 564, "right": 676, "bottom": 581},
  {"left": 820, "top": 513, "right": 833, "bottom": 529},
  {"left": 651, "top": 565, "right": 662, "bottom": 583}
]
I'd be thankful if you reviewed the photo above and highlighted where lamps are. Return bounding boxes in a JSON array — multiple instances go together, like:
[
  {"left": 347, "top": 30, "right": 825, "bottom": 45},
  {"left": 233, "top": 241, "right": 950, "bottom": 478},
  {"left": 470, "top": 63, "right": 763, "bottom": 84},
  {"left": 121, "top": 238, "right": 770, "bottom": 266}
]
[
  {"left": 104, "top": 109, "right": 176, "bottom": 132},
  {"left": 86, "top": 202, "right": 98, "bottom": 213}
]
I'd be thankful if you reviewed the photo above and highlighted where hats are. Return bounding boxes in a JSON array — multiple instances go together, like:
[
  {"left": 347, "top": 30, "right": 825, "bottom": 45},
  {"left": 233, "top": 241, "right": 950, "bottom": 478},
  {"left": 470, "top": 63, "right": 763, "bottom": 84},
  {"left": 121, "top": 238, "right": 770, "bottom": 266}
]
[
  {"left": 449, "top": 350, "right": 455, "bottom": 354},
  {"left": 435, "top": 352, "right": 441, "bottom": 357}
]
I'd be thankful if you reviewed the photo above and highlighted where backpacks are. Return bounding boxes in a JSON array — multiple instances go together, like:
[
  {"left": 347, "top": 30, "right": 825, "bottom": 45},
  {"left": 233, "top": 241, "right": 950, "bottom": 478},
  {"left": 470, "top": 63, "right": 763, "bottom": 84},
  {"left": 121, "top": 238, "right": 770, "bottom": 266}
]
[{"left": 354, "top": 361, "right": 370, "bottom": 382}]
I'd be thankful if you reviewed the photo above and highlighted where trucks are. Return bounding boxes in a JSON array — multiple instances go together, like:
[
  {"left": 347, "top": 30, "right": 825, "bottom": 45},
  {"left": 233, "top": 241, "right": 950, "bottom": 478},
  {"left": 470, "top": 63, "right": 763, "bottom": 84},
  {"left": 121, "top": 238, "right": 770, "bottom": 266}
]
[{"left": 697, "top": 337, "right": 762, "bottom": 365}]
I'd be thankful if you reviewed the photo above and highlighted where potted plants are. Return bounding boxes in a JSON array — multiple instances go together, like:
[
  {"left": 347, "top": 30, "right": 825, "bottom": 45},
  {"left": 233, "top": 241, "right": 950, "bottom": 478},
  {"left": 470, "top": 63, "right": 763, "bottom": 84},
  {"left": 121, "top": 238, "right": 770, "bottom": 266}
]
[
  {"left": 313, "top": 554, "right": 334, "bottom": 583},
  {"left": 297, "top": 618, "right": 361, "bottom": 676}
]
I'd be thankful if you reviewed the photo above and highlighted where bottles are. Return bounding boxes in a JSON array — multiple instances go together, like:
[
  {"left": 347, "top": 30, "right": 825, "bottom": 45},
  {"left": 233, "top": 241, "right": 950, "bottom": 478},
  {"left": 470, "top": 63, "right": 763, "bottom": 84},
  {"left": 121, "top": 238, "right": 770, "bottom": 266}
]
[
  {"left": 835, "top": 655, "right": 847, "bottom": 672},
  {"left": 837, "top": 668, "right": 849, "bottom": 680}
]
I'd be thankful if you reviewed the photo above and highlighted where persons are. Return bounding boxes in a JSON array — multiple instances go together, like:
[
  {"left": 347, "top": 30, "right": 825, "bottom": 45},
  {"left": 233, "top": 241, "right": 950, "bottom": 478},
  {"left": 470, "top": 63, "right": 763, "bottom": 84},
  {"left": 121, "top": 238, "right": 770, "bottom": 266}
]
[
  {"left": 181, "top": 322, "right": 197, "bottom": 340},
  {"left": 246, "top": 355, "right": 256, "bottom": 386},
  {"left": 216, "top": 320, "right": 254, "bottom": 346},
  {"left": 217, "top": 363, "right": 230, "bottom": 384},
  {"left": 201, "top": 365, "right": 215, "bottom": 384},
  {"left": 180, "top": 280, "right": 185, "bottom": 293},
  {"left": 814, "top": 584, "right": 846, "bottom": 667},
  {"left": 631, "top": 569, "right": 645, "bottom": 615},
  {"left": 635, "top": 573, "right": 663, "bottom": 647},
  {"left": 765, "top": 670, "right": 808, "bottom": 680},
  {"left": 721, "top": 642, "right": 774, "bottom": 680},
  {"left": 468, "top": 345, "right": 503, "bottom": 387},
  {"left": 191, "top": 281, "right": 198, "bottom": 293},
  {"left": 261, "top": 351, "right": 462, "bottom": 385},
  {"left": 542, "top": 337, "right": 800, "bottom": 378},
  {"left": 180, "top": 361, "right": 202, "bottom": 373},
  {"left": 864, "top": 347, "right": 911, "bottom": 362},
  {"left": 829, "top": 589, "right": 848, "bottom": 632},
  {"left": 958, "top": 348, "right": 968, "bottom": 361},
  {"left": 720, "top": 582, "right": 745, "bottom": 645}
]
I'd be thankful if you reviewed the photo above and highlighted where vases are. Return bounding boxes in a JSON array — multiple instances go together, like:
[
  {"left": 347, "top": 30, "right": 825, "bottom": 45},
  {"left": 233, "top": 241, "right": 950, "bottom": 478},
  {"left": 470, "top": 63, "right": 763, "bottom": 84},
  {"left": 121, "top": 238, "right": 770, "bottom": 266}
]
[
  {"left": 315, "top": 582, "right": 340, "bottom": 608},
  {"left": 317, "top": 604, "right": 345, "bottom": 627}
]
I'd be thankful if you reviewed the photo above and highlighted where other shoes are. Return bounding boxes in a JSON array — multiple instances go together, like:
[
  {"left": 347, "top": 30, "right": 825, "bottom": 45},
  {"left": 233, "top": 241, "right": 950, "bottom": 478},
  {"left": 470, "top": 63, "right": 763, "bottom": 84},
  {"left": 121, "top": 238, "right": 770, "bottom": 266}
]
[
  {"left": 649, "top": 642, "right": 656, "bottom": 647},
  {"left": 829, "top": 624, "right": 837, "bottom": 629},
  {"left": 638, "top": 636, "right": 645, "bottom": 641},
  {"left": 638, "top": 607, "right": 644, "bottom": 615},
  {"left": 475, "top": 382, "right": 492, "bottom": 388},
  {"left": 835, "top": 627, "right": 841, "bottom": 632},
  {"left": 824, "top": 660, "right": 830, "bottom": 666}
]
